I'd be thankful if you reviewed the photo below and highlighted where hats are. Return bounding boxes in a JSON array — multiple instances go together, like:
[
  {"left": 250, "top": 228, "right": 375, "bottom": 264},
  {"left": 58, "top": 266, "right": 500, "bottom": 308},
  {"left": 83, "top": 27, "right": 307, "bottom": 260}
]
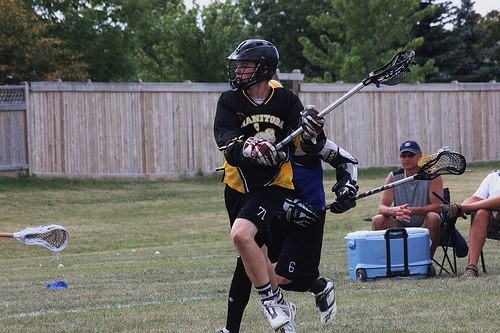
[{"left": 400, "top": 140, "right": 421, "bottom": 155}]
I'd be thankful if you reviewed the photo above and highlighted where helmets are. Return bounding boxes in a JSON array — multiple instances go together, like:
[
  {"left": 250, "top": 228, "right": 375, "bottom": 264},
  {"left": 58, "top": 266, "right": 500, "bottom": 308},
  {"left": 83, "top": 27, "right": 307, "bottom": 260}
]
[{"left": 227, "top": 40, "right": 279, "bottom": 89}]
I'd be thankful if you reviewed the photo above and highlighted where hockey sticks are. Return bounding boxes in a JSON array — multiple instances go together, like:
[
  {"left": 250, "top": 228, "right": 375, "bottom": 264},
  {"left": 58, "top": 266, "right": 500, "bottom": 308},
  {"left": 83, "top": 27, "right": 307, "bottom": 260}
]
[
  {"left": 0, "top": 225, "right": 71, "bottom": 252},
  {"left": 316, "top": 146, "right": 467, "bottom": 211},
  {"left": 276, "top": 48, "right": 416, "bottom": 148}
]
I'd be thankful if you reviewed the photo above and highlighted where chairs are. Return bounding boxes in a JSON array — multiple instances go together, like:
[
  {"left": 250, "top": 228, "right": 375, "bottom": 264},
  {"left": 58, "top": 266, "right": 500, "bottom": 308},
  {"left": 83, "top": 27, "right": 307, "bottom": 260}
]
[{"left": 429, "top": 186, "right": 500, "bottom": 278}]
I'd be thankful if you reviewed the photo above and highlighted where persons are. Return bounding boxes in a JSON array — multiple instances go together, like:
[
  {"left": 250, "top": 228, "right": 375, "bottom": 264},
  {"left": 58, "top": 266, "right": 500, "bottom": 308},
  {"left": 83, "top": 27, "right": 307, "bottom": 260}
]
[
  {"left": 214, "top": 38, "right": 326, "bottom": 333},
  {"left": 220, "top": 78, "right": 359, "bottom": 333},
  {"left": 372, "top": 140, "right": 443, "bottom": 276},
  {"left": 442, "top": 169, "right": 500, "bottom": 279}
]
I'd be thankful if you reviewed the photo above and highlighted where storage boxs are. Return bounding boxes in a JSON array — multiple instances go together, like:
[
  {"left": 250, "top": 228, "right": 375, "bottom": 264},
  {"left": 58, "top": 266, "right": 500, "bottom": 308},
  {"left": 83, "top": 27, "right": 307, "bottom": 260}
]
[{"left": 346, "top": 227, "right": 434, "bottom": 283}]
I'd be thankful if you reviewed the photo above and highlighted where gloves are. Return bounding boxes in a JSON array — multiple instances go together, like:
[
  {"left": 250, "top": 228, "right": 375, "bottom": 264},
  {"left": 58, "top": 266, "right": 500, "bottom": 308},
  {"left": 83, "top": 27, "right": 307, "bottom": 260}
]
[
  {"left": 330, "top": 181, "right": 359, "bottom": 214},
  {"left": 300, "top": 105, "right": 326, "bottom": 140},
  {"left": 243, "top": 137, "right": 287, "bottom": 167},
  {"left": 279, "top": 196, "right": 321, "bottom": 227}
]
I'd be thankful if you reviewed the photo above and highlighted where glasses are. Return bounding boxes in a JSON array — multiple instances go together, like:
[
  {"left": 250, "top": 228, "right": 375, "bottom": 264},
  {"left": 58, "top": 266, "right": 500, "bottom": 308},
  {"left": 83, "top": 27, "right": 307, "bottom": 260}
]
[{"left": 402, "top": 152, "right": 415, "bottom": 158}]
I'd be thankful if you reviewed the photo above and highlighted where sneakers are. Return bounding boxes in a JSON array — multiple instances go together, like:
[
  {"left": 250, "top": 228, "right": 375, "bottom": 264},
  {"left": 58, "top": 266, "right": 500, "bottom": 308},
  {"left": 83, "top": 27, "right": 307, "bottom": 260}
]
[
  {"left": 459, "top": 264, "right": 479, "bottom": 281},
  {"left": 313, "top": 278, "right": 337, "bottom": 327},
  {"left": 261, "top": 300, "right": 297, "bottom": 333},
  {"left": 440, "top": 203, "right": 461, "bottom": 226}
]
